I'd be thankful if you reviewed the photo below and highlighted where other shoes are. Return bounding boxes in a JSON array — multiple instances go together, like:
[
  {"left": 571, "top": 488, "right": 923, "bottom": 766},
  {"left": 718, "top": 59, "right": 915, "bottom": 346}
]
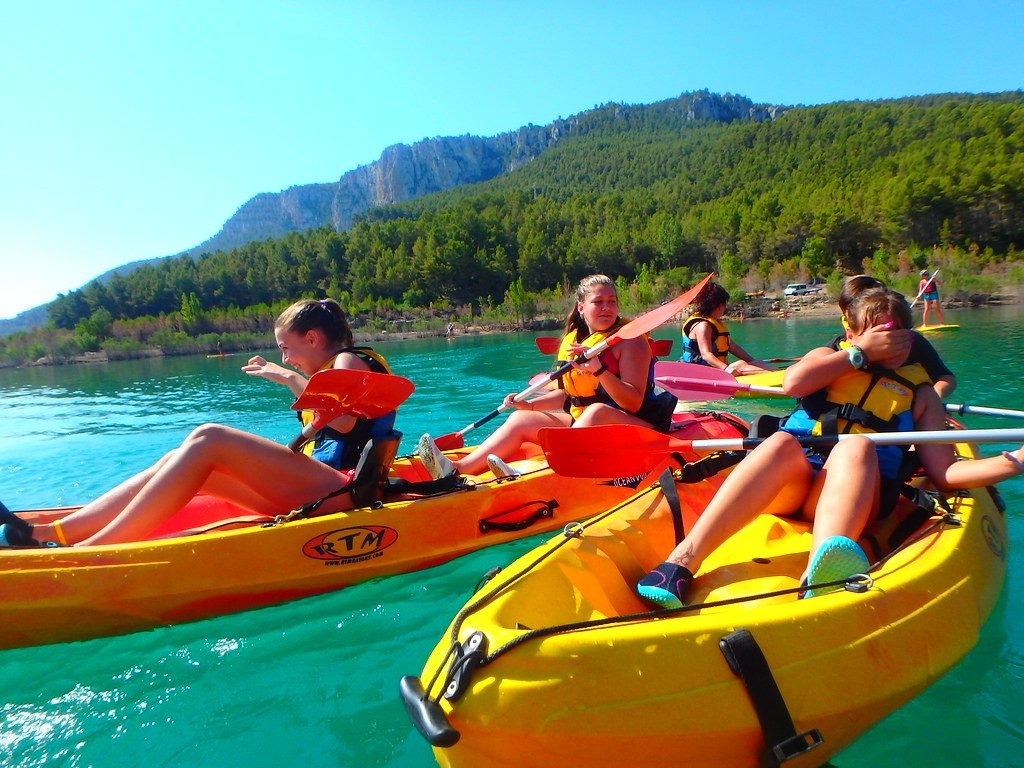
[
  {"left": 636, "top": 561, "right": 697, "bottom": 610},
  {"left": 0, "top": 500, "right": 30, "bottom": 527},
  {"left": 486, "top": 453, "right": 520, "bottom": 478},
  {"left": 798, "top": 535, "right": 873, "bottom": 599},
  {"left": 417, "top": 432, "right": 454, "bottom": 480},
  {"left": 0, "top": 522, "right": 57, "bottom": 548}
]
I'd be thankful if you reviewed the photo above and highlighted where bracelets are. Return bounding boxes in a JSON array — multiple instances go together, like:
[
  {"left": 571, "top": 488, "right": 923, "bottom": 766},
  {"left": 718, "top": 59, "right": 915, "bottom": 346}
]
[
  {"left": 593, "top": 363, "right": 608, "bottom": 377},
  {"left": 531, "top": 401, "right": 535, "bottom": 411},
  {"left": 1001, "top": 449, "right": 1024, "bottom": 474}
]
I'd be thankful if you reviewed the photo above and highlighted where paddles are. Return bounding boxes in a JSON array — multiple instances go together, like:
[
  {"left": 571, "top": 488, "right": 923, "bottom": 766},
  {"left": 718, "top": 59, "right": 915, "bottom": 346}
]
[
  {"left": 758, "top": 357, "right": 803, "bottom": 362},
  {"left": 285, "top": 366, "right": 416, "bottom": 452},
  {"left": 649, "top": 358, "right": 1024, "bottom": 425},
  {"left": 535, "top": 424, "right": 1024, "bottom": 478},
  {"left": 430, "top": 272, "right": 717, "bottom": 452}
]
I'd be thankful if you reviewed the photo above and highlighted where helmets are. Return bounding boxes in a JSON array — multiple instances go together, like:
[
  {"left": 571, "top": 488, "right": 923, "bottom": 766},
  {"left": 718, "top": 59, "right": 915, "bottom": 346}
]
[{"left": 920, "top": 269, "right": 929, "bottom": 275}]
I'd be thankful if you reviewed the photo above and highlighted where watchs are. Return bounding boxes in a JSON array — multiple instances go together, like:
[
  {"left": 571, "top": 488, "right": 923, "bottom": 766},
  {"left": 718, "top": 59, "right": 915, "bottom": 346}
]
[{"left": 839, "top": 341, "right": 866, "bottom": 371}]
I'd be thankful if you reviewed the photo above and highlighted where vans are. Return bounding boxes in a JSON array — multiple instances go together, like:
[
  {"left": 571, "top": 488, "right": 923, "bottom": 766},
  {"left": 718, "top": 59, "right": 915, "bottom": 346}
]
[{"left": 783, "top": 283, "right": 807, "bottom": 296}]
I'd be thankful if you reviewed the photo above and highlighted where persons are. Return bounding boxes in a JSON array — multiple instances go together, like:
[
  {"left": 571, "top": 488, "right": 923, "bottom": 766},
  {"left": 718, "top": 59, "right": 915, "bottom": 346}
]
[
  {"left": 634, "top": 264, "right": 1024, "bottom": 611},
  {"left": 0, "top": 297, "right": 397, "bottom": 549},
  {"left": 784, "top": 311, "right": 787, "bottom": 318},
  {"left": 418, "top": 275, "right": 655, "bottom": 483}
]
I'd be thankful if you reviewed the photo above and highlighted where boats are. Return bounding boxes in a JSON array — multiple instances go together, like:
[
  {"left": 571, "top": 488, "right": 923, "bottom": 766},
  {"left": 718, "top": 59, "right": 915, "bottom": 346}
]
[
  {"left": 529, "top": 359, "right": 789, "bottom": 403},
  {"left": 395, "top": 408, "right": 1009, "bottom": 768},
  {"left": 841, "top": 315, "right": 962, "bottom": 332},
  {"left": 0, "top": 407, "right": 753, "bottom": 651}
]
